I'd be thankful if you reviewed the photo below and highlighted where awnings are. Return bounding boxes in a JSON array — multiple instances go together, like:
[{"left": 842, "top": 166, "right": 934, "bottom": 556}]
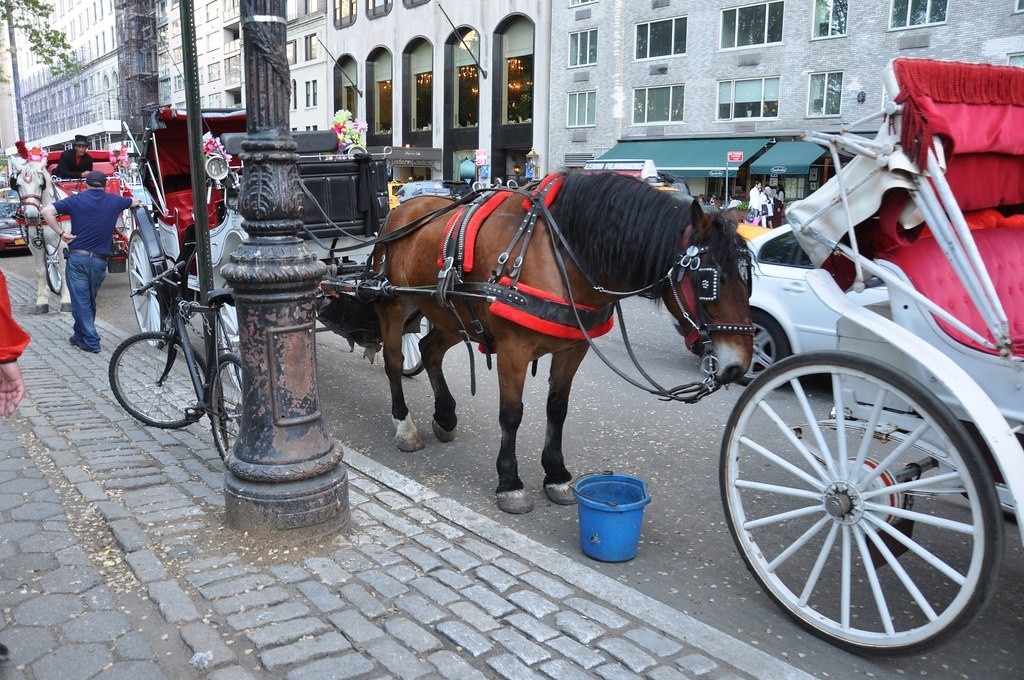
[
  {"left": 752, "top": 140, "right": 827, "bottom": 176},
  {"left": 581, "top": 135, "right": 771, "bottom": 178}
]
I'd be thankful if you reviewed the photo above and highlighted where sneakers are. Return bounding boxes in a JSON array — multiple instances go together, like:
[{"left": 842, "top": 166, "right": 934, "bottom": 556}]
[{"left": 68, "top": 334, "right": 101, "bottom": 354}]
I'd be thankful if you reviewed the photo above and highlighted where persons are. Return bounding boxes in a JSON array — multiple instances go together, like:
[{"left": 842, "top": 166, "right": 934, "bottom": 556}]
[
  {"left": 697, "top": 193, "right": 721, "bottom": 207},
  {"left": 0, "top": 269, "right": 32, "bottom": 419},
  {"left": 748, "top": 179, "right": 785, "bottom": 230},
  {"left": 728, "top": 194, "right": 740, "bottom": 209},
  {"left": 40, "top": 170, "right": 140, "bottom": 354},
  {"left": 51, "top": 134, "right": 93, "bottom": 178}
]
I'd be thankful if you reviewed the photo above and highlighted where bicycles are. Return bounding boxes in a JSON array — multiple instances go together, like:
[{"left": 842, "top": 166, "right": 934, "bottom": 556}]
[{"left": 108, "top": 260, "right": 242, "bottom": 462}]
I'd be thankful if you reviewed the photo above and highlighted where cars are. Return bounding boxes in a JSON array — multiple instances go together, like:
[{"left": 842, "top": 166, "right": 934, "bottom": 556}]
[
  {"left": 734, "top": 222, "right": 837, "bottom": 386},
  {"left": 0, "top": 201, "right": 27, "bottom": 253}
]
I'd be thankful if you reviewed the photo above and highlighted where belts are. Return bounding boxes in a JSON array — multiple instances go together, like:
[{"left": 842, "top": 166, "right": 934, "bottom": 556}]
[{"left": 68, "top": 248, "right": 110, "bottom": 263}]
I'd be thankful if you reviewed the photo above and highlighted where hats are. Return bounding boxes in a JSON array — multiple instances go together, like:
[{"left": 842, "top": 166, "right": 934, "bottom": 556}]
[
  {"left": 73, "top": 135, "right": 89, "bottom": 147},
  {"left": 86, "top": 171, "right": 106, "bottom": 188}
]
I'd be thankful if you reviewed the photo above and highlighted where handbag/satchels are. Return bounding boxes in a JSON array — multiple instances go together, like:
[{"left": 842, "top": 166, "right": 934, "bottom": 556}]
[{"left": 758, "top": 204, "right": 774, "bottom": 217}]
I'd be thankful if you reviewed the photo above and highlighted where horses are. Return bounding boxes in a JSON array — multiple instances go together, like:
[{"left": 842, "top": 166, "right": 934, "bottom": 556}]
[
  {"left": 358, "top": 169, "right": 758, "bottom": 514},
  {"left": 9, "top": 153, "right": 81, "bottom": 315}
]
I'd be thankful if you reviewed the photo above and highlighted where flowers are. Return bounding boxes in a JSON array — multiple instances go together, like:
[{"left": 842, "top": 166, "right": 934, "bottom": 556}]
[
  {"left": 28, "top": 146, "right": 48, "bottom": 162},
  {"left": 110, "top": 144, "right": 130, "bottom": 169},
  {"left": 203, "top": 131, "right": 232, "bottom": 164},
  {"left": 329, "top": 108, "right": 370, "bottom": 154}
]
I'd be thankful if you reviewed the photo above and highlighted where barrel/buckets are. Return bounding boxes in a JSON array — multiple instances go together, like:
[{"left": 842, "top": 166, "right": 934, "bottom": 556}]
[
  {"left": 108, "top": 251, "right": 126, "bottom": 273},
  {"left": 573, "top": 473, "right": 651, "bottom": 563}
]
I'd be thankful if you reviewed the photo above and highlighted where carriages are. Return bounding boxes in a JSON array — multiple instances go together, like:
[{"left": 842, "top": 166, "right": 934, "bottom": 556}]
[
  {"left": 6, "top": 149, "right": 129, "bottom": 315},
  {"left": 127, "top": 107, "right": 757, "bottom": 515}
]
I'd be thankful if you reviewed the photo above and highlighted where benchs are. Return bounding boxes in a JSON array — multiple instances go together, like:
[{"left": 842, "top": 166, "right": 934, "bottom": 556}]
[
  {"left": 48, "top": 162, "right": 115, "bottom": 180},
  {"left": 166, "top": 185, "right": 221, "bottom": 247},
  {"left": 872, "top": 207, "right": 1024, "bottom": 357}
]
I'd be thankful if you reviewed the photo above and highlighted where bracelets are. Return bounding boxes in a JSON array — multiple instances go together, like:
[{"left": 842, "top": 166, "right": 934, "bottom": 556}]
[{"left": 58, "top": 230, "right": 65, "bottom": 237}]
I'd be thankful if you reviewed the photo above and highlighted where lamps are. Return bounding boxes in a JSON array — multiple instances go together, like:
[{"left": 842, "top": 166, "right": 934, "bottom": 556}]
[{"left": 526, "top": 147, "right": 540, "bottom": 180}]
